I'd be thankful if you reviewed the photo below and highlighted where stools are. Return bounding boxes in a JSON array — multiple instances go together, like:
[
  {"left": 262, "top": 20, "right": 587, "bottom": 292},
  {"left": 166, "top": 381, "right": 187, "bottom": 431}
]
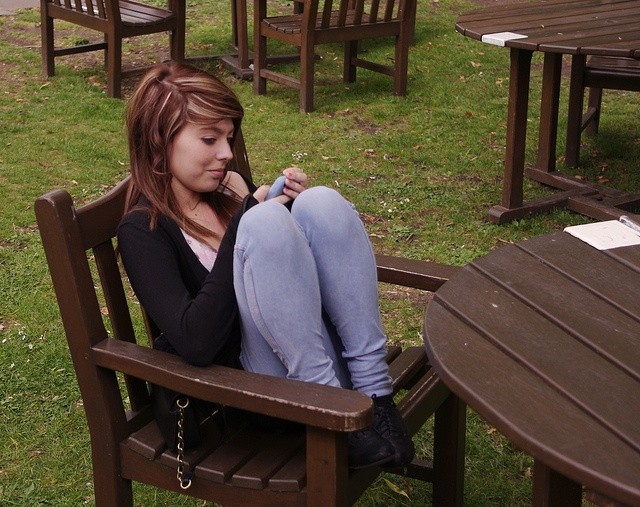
[{"left": 564, "top": 57, "right": 639, "bottom": 172}]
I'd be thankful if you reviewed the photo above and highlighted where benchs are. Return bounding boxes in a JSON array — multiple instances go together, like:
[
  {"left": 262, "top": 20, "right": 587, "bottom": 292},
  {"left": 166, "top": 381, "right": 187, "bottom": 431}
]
[
  {"left": 253, "top": 0, "right": 416, "bottom": 114},
  {"left": 40, "top": 0, "right": 186, "bottom": 98}
]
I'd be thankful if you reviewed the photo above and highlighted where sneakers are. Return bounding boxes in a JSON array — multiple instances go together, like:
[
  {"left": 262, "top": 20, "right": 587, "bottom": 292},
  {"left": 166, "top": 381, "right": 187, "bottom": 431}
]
[
  {"left": 371, "top": 391, "right": 416, "bottom": 468},
  {"left": 298, "top": 420, "right": 400, "bottom": 475}
]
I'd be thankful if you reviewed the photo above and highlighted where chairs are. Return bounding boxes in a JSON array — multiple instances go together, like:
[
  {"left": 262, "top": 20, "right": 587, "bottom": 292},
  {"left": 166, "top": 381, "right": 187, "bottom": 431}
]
[{"left": 34, "top": 124, "right": 467, "bottom": 507}]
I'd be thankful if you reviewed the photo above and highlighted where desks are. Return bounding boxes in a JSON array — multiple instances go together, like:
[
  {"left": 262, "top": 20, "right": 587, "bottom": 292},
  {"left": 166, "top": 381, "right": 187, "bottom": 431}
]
[
  {"left": 169, "top": 0, "right": 319, "bottom": 79},
  {"left": 454, "top": 0, "right": 638, "bottom": 226},
  {"left": 425, "top": 218, "right": 636, "bottom": 507}
]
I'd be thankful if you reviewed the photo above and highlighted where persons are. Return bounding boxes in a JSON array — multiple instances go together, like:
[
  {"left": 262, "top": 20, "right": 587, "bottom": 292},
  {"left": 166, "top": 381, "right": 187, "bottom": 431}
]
[{"left": 114, "top": 59, "right": 415, "bottom": 481}]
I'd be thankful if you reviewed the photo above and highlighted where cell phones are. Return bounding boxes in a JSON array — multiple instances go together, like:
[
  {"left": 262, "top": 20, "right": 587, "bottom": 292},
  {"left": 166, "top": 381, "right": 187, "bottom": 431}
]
[{"left": 265, "top": 176, "right": 285, "bottom": 201}]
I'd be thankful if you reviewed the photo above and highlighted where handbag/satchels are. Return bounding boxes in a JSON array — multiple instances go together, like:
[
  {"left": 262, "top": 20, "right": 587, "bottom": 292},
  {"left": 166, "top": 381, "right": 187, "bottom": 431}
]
[{"left": 145, "top": 332, "right": 202, "bottom": 456}]
